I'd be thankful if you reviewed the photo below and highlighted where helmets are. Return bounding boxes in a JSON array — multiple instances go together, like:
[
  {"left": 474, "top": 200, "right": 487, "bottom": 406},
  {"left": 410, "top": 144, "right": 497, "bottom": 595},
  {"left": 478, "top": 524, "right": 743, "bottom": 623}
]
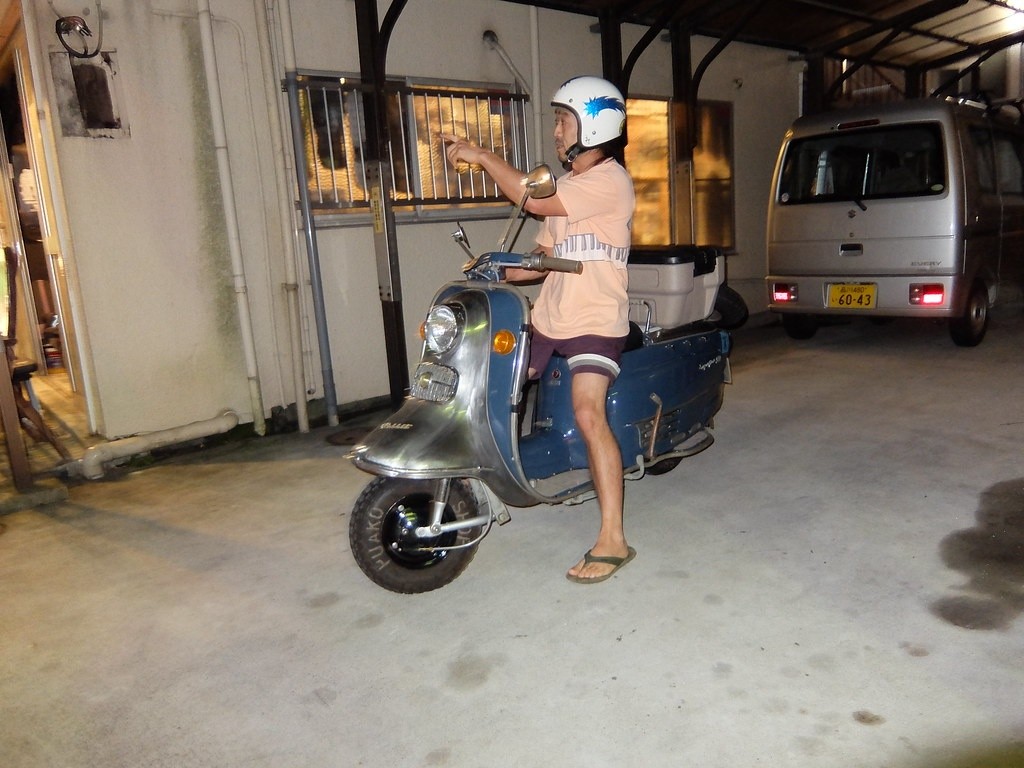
[{"left": 552, "top": 75, "right": 626, "bottom": 149}]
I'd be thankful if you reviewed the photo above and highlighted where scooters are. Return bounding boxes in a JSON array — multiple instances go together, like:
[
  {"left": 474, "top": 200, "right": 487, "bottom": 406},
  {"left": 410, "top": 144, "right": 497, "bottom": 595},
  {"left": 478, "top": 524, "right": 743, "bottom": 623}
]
[{"left": 348, "top": 164, "right": 748, "bottom": 595}]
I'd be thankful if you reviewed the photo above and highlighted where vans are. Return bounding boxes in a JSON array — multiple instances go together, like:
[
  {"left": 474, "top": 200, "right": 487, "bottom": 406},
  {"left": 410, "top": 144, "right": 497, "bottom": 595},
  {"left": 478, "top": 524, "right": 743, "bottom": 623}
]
[{"left": 765, "top": 95, "right": 1023, "bottom": 346}]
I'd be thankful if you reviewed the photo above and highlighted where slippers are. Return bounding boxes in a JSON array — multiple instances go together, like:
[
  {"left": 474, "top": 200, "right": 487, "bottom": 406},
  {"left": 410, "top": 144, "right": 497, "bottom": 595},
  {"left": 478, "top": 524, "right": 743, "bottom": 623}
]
[{"left": 565, "top": 542, "right": 636, "bottom": 584}]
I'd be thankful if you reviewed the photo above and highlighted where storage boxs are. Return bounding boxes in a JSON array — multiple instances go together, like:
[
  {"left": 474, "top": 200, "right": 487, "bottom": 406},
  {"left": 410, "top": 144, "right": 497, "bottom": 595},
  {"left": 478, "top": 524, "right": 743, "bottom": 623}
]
[{"left": 627, "top": 243, "right": 726, "bottom": 330}]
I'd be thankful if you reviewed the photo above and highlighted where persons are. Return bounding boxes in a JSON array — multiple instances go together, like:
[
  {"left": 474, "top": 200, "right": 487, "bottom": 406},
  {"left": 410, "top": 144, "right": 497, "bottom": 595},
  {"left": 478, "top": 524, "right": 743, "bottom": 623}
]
[{"left": 437, "top": 75, "right": 637, "bottom": 583}]
